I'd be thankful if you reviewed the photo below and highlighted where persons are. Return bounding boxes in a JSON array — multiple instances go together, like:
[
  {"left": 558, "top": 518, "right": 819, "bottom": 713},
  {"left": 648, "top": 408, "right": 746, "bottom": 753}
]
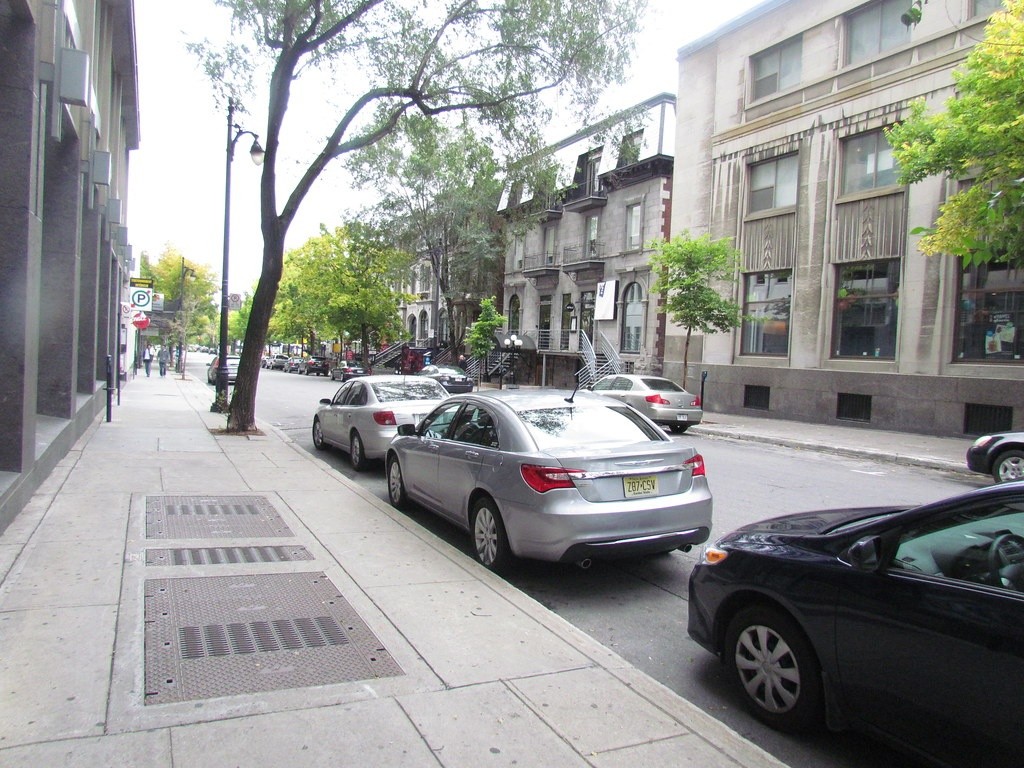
[
  {"left": 458, "top": 355, "right": 467, "bottom": 371},
  {"left": 157, "top": 345, "right": 169, "bottom": 376},
  {"left": 143, "top": 344, "right": 154, "bottom": 377}
]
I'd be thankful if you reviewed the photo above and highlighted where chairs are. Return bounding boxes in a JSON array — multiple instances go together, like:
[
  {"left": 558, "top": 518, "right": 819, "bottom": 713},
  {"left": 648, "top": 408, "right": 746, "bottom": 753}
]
[{"left": 468, "top": 409, "right": 496, "bottom": 445}]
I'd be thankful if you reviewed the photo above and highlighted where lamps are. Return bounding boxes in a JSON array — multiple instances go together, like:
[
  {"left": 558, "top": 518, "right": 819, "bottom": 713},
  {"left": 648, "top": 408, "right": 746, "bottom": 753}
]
[{"left": 566, "top": 303, "right": 575, "bottom": 316}]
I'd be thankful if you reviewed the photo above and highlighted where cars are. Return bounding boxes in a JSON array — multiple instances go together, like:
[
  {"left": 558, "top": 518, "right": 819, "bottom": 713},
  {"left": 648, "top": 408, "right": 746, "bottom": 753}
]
[
  {"left": 384, "top": 389, "right": 712, "bottom": 578},
  {"left": 312, "top": 375, "right": 459, "bottom": 471},
  {"left": 266, "top": 354, "right": 289, "bottom": 371},
  {"left": 205, "top": 355, "right": 241, "bottom": 385},
  {"left": 284, "top": 356, "right": 304, "bottom": 373},
  {"left": 685, "top": 480, "right": 1023, "bottom": 764},
  {"left": 330, "top": 361, "right": 370, "bottom": 382},
  {"left": 417, "top": 365, "right": 474, "bottom": 393},
  {"left": 260, "top": 355, "right": 270, "bottom": 368},
  {"left": 298, "top": 356, "right": 331, "bottom": 376},
  {"left": 186, "top": 344, "right": 218, "bottom": 355},
  {"left": 966, "top": 431, "right": 1024, "bottom": 484},
  {"left": 584, "top": 373, "right": 704, "bottom": 434}
]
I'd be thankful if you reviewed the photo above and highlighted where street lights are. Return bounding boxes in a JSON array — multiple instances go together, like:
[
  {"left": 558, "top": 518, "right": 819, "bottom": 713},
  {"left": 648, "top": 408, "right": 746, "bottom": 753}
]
[
  {"left": 176, "top": 256, "right": 196, "bottom": 373},
  {"left": 504, "top": 334, "right": 523, "bottom": 384},
  {"left": 209, "top": 95, "right": 266, "bottom": 413}
]
